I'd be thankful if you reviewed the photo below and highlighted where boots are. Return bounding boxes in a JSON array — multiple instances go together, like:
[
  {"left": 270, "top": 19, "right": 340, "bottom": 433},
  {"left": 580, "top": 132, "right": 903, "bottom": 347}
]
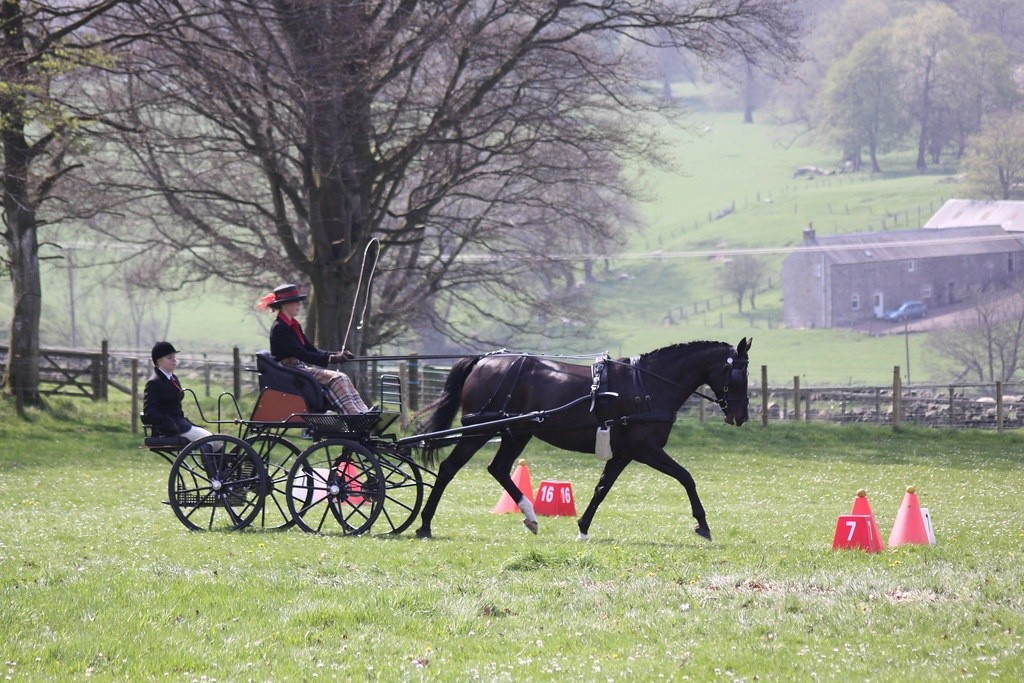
[
  {"left": 200, "top": 444, "right": 233, "bottom": 494},
  {"left": 214, "top": 445, "right": 233, "bottom": 488}
]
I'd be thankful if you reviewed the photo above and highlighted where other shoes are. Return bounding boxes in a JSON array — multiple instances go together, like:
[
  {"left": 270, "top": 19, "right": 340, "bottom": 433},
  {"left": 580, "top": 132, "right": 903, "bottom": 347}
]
[
  {"left": 363, "top": 404, "right": 379, "bottom": 427},
  {"left": 348, "top": 405, "right": 375, "bottom": 432}
]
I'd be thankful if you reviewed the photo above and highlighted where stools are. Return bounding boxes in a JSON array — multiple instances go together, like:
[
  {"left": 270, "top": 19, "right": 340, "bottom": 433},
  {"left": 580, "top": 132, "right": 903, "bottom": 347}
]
[{"left": 140, "top": 412, "right": 202, "bottom": 452}]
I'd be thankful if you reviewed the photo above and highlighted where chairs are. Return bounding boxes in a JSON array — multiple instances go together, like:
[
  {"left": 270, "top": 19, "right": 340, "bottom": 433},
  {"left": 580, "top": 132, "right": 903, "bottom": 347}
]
[{"left": 255, "top": 351, "right": 332, "bottom": 428}]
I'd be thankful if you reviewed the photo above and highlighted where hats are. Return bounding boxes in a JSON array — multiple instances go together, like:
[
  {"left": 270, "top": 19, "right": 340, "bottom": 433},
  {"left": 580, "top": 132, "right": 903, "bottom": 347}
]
[
  {"left": 151, "top": 342, "right": 180, "bottom": 366},
  {"left": 256, "top": 284, "right": 309, "bottom": 312}
]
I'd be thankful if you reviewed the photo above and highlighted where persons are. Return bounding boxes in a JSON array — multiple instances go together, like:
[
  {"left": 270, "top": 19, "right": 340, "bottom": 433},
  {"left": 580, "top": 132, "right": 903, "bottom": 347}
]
[
  {"left": 141, "top": 342, "right": 249, "bottom": 492},
  {"left": 270, "top": 288, "right": 380, "bottom": 433}
]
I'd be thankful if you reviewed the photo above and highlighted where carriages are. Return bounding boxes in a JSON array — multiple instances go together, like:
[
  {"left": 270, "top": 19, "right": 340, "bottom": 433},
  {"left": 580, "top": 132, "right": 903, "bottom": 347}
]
[{"left": 137, "top": 331, "right": 755, "bottom": 547}]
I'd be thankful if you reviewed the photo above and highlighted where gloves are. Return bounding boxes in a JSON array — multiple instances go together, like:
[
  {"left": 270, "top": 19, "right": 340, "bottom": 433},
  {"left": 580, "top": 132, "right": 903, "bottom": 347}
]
[
  {"left": 329, "top": 354, "right": 347, "bottom": 363},
  {"left": 337, "top": 350, "right": 355, "bottom": 359}
]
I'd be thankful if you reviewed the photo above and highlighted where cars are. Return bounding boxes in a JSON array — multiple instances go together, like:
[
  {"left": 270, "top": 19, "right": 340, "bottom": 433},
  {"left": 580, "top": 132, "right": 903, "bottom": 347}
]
[{"left": 884, "top": 299, "right": 928, "bottom": 322}]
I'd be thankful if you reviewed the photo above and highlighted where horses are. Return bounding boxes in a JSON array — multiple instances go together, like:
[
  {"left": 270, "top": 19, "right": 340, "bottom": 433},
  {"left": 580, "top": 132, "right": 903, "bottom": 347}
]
[{"left": 405, "top": 336, "right": 753, "bottom": 543}]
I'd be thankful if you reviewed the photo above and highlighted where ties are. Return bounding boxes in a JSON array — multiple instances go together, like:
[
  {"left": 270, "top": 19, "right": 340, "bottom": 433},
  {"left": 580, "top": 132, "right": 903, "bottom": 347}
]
[{"left": 170, "top": 375, "right": 182, "bottom": 392}]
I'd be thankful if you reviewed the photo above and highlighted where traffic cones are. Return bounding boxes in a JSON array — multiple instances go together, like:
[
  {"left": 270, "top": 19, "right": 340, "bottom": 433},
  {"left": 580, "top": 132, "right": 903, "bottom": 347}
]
[
  {"left": 492, "top": 456, "right": 533, "bottom": 513},
  {"left": 848, "top": 488, "right": 884, "bottom": 554},
  {"left": 885, "top": 480, "right": 930, "bottom": 549},
  {"left": 330, "top": 445, "right": 372, "bottom": 506}
]
